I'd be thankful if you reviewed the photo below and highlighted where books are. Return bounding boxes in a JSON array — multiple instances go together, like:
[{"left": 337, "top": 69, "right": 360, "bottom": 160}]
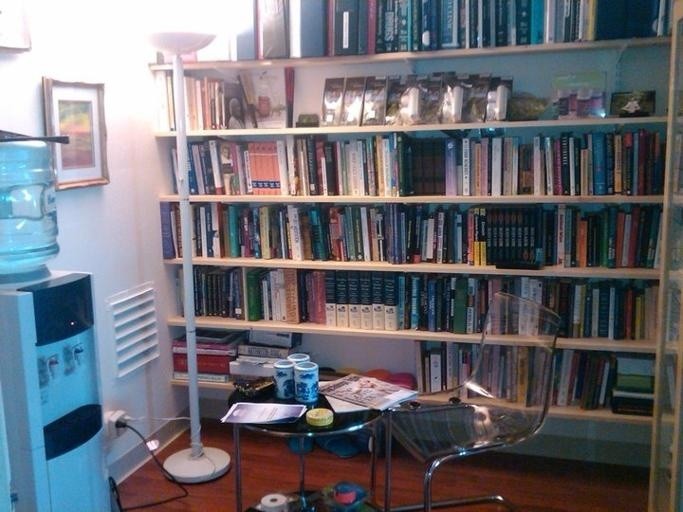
[
  {"left": 178, "top": 267, "right": 660, "bottom": 340},
  {"left": 167, "top": 69, "right": 602, "bottom": 131},
  {"left": 234, "top": 0, "right": 671, "bottom": 59},
  {"left": 173, "top": 131, "right": 666, "bottom": 196},
  {"left": 172, "top": 327, "right": 676, "bottom": 417},
  {"left": 172, "top": 202, "right": 664, "bottom": 270}
]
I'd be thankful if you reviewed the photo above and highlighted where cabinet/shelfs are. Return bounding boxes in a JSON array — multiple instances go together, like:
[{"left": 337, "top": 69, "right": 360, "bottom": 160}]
[{"left": 148, "top": 1, "right": 681, "bottom": 426}]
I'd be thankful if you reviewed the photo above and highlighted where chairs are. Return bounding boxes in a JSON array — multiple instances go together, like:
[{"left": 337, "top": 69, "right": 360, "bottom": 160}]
[{"left": 370, "top": 291, "right": 562, "bottom": 512}]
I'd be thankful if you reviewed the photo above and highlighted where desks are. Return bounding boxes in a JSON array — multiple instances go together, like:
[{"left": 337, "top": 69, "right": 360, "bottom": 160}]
[{"left": 227, "top": 378, "right": 386, "bottom": 512}]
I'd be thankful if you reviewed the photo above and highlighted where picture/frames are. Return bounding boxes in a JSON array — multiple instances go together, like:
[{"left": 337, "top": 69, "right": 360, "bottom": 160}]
[{"left": 42, "top": 75, "right": 110, "bottom": 190}]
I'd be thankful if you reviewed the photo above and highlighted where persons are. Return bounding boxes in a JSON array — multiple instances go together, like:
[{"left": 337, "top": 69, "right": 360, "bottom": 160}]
[{"left": 623, "top": 96, "right": 640, "bottom": 112}]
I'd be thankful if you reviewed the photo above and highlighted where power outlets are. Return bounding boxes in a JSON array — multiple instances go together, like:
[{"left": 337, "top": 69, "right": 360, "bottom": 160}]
[{"left": 111, "top": 410, "right": 132, "bottom": 437}]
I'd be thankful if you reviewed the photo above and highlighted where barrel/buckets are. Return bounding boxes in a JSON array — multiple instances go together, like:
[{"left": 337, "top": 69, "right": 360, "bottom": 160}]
[{"left": 0, "top": 140, "right": 60, "bottom": 274}]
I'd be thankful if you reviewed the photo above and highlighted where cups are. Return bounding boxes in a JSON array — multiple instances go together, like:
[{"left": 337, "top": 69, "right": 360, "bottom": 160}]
[{"left": 272, "top": 354, "right": 319, "bottom": 404}]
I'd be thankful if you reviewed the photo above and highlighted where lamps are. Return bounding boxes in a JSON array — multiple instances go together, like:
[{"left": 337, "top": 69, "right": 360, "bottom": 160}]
[{"left": 149, "top": 32, "right": 231, "bottom": 484}]
